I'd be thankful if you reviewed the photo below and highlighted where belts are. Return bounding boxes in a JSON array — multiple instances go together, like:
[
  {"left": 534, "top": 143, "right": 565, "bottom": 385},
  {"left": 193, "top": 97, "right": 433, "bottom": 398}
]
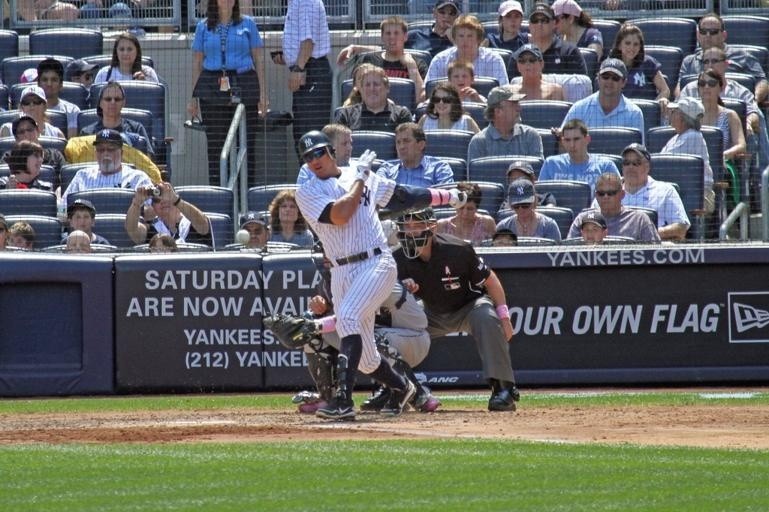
[{"left": 330, "top": 248, "right": 382, "bottom": 267}]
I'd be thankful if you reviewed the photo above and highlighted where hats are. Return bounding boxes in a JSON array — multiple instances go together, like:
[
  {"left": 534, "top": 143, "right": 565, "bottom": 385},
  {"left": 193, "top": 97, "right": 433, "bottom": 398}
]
[
  {"left": 514, "top": 44, "right": 543, "bottom": 60},
  {"left": 508, "top": 161, "right": 536, "bottom": 206},
  {"left": 67, "top": 199, "right": 95, "bottom": 211},
  {"left": 598, "top": 59, "right": 628, "bottom": 77},
  {"left": 622, "top": 143, "right": 650, "bottom": 161},
  {"left": 580, "top": 215, "right": 608, "bottom": 229},
  {"left": 21, "top": 68, "right": 38, "bottom": 83},
  {"left": 435, "top": 0, "right": 461, "bottom": 11},
  {"left": 668, "top": 97, "right": 705, "bottom": 120},
  {"left": 19, "top": 86, "right": 48, "bottom": 103},
  {"left": 499, "top": 0, "right": 581, "bottom": 19},
  {"left": 93, "top": 128, "right": 123, "bottom": 144},
  {"left": 239, "top": 211, "right": 268, "bottom": 228},
  {"left": 487, "top": 87, "right": 526, "bottom": 104},
  {"left": 67, "top": 60, "right": 98, "bottom": 75}
]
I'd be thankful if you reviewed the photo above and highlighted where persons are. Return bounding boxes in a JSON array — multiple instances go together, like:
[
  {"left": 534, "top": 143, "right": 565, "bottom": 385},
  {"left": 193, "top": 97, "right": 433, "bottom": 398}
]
[
  {"left": 293, "top": 129, "right": 469, "bottom": 421},
  {"left": 0, "top": 2, "right": 767, "bottom": 255},
  {"left": 392, "top": 207, "right": 521, "bottom": 411},
  {"left": 262, "top": 239, "right": 442, "bottom": 413}
]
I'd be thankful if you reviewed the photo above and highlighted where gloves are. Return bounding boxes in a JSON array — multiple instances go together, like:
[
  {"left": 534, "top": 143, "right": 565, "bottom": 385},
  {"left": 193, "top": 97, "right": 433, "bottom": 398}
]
[
  {"left": 449, "top": 187, "right": 467, "bottom": 208},
  {"left": 354, "top": 148, "right": 377, "bottom": 183}
]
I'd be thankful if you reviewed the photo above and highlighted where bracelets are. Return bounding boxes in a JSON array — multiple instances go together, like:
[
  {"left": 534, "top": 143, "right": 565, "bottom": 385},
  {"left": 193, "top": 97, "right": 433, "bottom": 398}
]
[
  {"left": 496, "top": 305, "right": 511, "bottom": 320},
  {"left": 321, "top": 315, "right": 336, "bottom": 334}
]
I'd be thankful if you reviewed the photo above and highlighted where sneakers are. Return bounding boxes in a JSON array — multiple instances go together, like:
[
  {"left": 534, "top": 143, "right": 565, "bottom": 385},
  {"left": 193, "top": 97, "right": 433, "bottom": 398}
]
[
  {"left": 293, "top": 379, "right": 441, "bottom": 421},
  {"left": 487, "top": 383, "right": 518, "bottom": 410}
]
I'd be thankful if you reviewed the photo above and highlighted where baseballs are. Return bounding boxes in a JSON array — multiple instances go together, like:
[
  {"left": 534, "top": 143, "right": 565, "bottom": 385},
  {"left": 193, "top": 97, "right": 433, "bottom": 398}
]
[{"left": 237, "top": 229, "right": 250, "bottom": 244}]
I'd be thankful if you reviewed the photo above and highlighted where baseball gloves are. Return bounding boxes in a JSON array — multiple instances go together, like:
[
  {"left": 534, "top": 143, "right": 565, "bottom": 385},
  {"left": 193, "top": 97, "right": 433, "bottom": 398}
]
[{"left": 263, "top": 314, "right": 316, "bottom": 349}]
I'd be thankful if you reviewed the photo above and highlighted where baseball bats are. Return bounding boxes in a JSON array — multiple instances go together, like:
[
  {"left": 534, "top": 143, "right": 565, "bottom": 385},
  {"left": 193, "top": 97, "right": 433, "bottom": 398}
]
[{"left": 379, "top": 192, "right": 464, "bottom": 221}]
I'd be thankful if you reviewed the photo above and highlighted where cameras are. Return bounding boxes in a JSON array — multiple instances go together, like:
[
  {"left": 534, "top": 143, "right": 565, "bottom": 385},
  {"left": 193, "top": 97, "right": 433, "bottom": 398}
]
[{"left": 146, "top": 186, "right": 161, "bottom": 196}]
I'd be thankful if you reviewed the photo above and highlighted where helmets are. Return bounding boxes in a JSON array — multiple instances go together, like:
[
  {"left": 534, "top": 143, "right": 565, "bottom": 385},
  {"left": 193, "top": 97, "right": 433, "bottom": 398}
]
[
  {"left": 396, "top": 207, "right": 437, "bottom": 259},
  {"left": 299, "top": 130, "right": 335, "bottom": 158}
]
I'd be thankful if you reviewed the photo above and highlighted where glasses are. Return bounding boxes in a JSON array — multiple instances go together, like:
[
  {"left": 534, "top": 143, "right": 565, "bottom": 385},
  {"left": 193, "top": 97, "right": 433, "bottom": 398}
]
[
  {"left": 117, "top": 47, "right": 136, "bottom": 52},
  {"left": 103, "top": 97, "right": 123, "bottom": 101},
  {"left": 601, "top": 72, "right": 621, "bottom": 81},
  {"left": 698, "top": 30, "right": 725, "bottom": 87},
  {"left": 439, "top": 9, "right": 458, "bottom": 16},
  {"left": 623, "top": 162, "right": 644, "bottom": 166},
  {"left": 513, "top": 204, "right": 533, "bottom": 211},
  {"left": 565, "top": 138, "right": 584, "bottom": 142},
  {"left": 531, "top": 17, "right": 551, "bottom": 26},
  {"left": 598, "top": 192, "right": 618, "bottom": 196},
  {"left": 22, "top": 100, "right": 41, "bottom": 104},
  {"left": 16, "top": 128, "right": 36, "bottom": 133},
  {"left": 95, "top": 148, "right": 116, "bottom": 152},
  {"left": 434, "top": 96, "right": 453, "bottom": 102},
  {"left": 244, "top": 229, "right": 264, "bottom": 234},
  {"left": 304, "top": 150, "right": 325, "bottom": 163}
]
